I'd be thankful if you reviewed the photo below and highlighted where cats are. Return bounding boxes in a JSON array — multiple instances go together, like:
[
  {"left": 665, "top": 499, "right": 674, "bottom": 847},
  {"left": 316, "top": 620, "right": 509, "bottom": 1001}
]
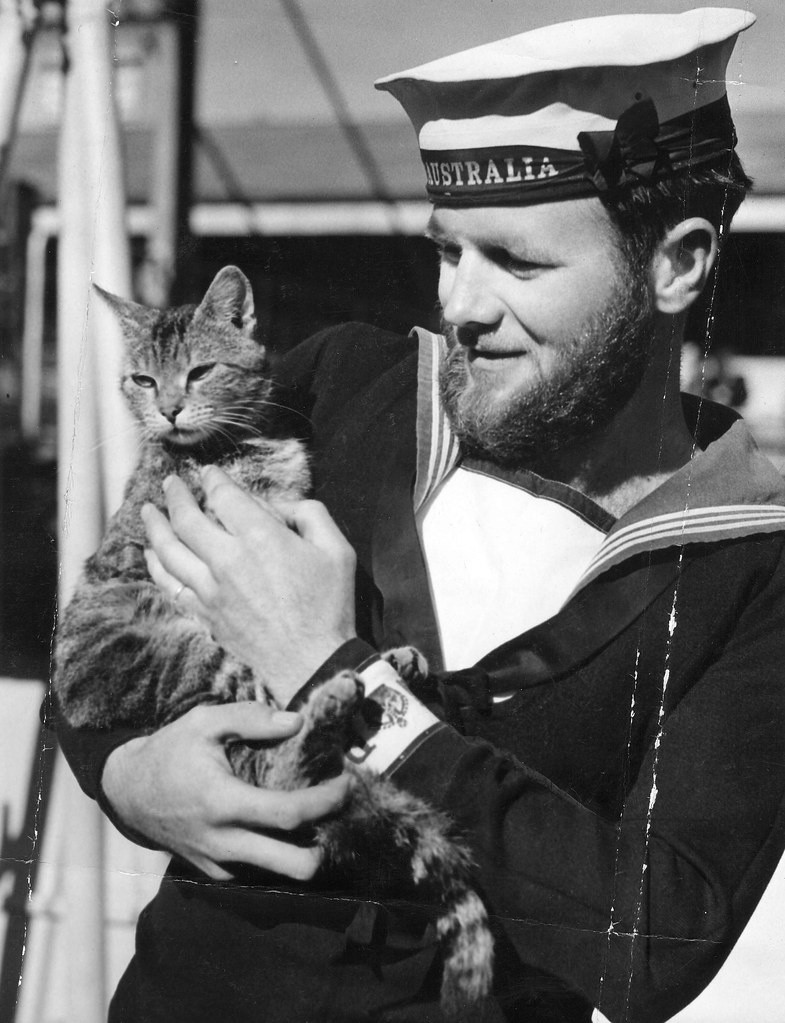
[{"left": 52, "top": 266, "right": 502, "bottom": 1023}]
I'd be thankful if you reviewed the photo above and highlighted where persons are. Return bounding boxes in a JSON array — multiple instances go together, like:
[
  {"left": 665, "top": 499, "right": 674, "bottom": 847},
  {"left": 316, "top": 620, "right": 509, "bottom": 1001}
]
[{"left": 40, "top": 8, "right": 785, "bottom": 1023}]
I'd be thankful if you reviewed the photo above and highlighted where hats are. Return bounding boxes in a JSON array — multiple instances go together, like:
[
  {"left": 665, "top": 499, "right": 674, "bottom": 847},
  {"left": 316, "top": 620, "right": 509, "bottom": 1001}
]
[{"left": 373, "top": 8, "right": 757, "bottom": 206}]
